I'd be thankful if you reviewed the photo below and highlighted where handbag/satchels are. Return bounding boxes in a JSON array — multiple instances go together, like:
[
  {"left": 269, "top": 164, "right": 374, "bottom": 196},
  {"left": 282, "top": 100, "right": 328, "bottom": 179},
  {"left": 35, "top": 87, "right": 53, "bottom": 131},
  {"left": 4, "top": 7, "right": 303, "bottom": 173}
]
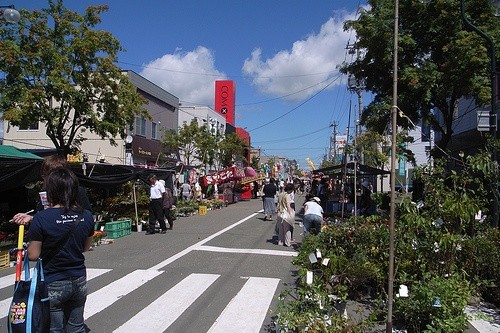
[{"left": 6, "top": 253, "right": 49, "bottom": 333}]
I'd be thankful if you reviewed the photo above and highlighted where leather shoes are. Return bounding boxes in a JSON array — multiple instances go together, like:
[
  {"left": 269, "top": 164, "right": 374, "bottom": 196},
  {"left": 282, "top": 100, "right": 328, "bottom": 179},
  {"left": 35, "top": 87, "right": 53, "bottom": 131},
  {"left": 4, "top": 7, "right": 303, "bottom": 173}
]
[
  {"left": 145, "top": 230, "right": 155, "bottom": 235},
  {"left": 160, "top": 230, "right": 166, "bottom": 234}
]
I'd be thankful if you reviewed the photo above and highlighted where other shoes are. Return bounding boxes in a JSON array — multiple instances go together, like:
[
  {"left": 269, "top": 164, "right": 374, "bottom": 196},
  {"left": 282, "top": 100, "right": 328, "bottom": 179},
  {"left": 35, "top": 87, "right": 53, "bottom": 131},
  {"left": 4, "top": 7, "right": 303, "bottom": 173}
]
[
  {"left": 291, "top": 239, "right": 296, "bottom": 243},
  {"left": 169, "top": 227, "right": 173, "bottom": 230}
]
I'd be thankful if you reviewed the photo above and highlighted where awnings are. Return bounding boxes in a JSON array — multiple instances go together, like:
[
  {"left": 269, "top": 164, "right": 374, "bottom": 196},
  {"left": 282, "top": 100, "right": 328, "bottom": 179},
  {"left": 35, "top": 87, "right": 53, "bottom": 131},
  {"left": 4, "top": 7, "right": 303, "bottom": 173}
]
[
  {"left": 310, "top": 162, "right": 390, "bottom": 175},
  {"left": 69, "top": 160, "right": 174, "bottom": 173},
  {"left": 0, "top": 145, "right": 45, "bottom": 161}
]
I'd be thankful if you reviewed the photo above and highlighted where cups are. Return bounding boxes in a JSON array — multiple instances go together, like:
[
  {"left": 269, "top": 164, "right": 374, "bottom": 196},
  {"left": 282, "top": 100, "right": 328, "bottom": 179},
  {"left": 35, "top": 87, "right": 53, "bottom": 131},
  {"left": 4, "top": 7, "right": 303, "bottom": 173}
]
[{"left": 39, "top": 192, "right": 49, "bottom": 205}]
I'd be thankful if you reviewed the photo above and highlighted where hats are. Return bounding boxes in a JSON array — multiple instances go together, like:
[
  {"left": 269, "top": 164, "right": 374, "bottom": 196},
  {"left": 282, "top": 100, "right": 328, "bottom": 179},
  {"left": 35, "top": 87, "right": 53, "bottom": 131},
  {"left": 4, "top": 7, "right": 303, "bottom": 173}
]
[{"left": 310, "top": 196, "right": 321, "bottom": 204}]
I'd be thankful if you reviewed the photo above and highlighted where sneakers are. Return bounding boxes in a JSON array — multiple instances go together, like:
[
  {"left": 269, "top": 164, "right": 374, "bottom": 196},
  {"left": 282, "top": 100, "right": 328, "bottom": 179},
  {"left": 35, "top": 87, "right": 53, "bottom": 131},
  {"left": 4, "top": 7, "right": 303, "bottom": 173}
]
[
  {"left": 269, "top": 218, "right": 272, "bottom": 220},
  {"left": 264, "top": 214, "right": 267, "bottom": 220}
]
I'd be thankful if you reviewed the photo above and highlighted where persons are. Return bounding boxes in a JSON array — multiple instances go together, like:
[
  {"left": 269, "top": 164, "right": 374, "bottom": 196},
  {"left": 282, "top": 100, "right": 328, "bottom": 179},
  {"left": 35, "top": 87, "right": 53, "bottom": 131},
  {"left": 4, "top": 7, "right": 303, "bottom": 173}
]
[
  {"left": 27, "top": 169, "right": 95, "bottom": 333},
  {"left": 143, "top": 175, "right": 167, "bottom": 235},
  {"left": 303, "top": 198, "right": 323, "bottom": 236},
  {"left": 158, "top": 179, "right": 173, "bottom": 230},
  {"left": 13, "top": 155, "right": 72, "bottom": 225},
  {"left": 175, "top": 174, "right": 349, "bottom": 221},
  {"left": 289, "top": 178, "right": 301, "bottom": 242},
  {"left": 277, "top": 183, "right": 294, "bottom": 247}
]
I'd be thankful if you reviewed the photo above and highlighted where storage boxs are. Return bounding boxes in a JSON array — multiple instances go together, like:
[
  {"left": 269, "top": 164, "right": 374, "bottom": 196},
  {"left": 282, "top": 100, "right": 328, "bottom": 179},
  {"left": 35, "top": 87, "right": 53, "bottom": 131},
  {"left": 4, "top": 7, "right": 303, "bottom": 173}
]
[{"left": 105, "top": 220, "right": 131, "bottom": 239}]
